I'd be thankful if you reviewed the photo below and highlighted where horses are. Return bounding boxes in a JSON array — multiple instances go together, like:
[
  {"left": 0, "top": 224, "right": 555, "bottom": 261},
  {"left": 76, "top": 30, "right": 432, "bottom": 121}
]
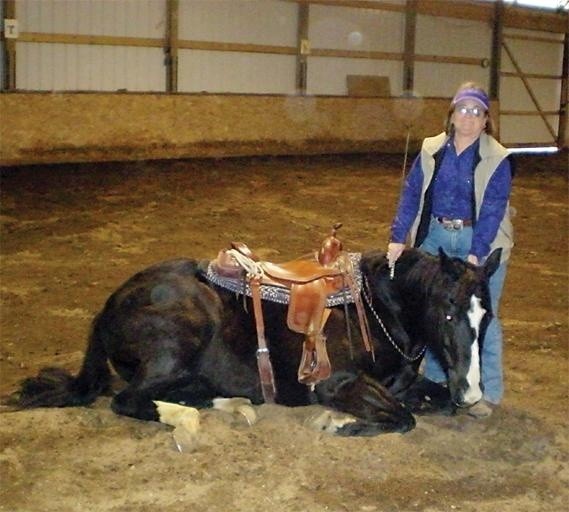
[{"left": 1, "top": 246, "right": 504, "bottom": 438}]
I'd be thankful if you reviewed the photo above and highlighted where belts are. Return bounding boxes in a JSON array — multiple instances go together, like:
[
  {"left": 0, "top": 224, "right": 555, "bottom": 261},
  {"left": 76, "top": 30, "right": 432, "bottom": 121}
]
[{"left": 433, "top": 215, "right": 473, "bottom": 231}]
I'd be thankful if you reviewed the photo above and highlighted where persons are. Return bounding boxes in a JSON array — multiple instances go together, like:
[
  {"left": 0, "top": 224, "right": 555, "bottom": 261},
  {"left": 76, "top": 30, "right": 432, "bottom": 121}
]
[{"left": 387, "top": 80, "right": 514, "bottom": 418}]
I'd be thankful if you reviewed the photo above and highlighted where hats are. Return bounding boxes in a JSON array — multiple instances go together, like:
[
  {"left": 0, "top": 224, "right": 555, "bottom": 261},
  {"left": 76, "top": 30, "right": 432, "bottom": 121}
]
[{"left": 453, "top": 81, "right": 489, "bottom": 110}]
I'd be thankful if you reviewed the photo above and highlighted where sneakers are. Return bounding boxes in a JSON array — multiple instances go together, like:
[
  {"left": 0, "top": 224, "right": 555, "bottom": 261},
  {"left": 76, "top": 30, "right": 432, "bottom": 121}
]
[{"left": 466, "top": 397, "right": 497, "bottom": 419}]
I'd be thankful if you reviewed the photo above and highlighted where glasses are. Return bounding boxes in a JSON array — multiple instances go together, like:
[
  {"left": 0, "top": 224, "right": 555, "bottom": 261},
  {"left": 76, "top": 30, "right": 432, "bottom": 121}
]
[{"left": 455, "top": 104, "right": 485, "bottom": 118}]
